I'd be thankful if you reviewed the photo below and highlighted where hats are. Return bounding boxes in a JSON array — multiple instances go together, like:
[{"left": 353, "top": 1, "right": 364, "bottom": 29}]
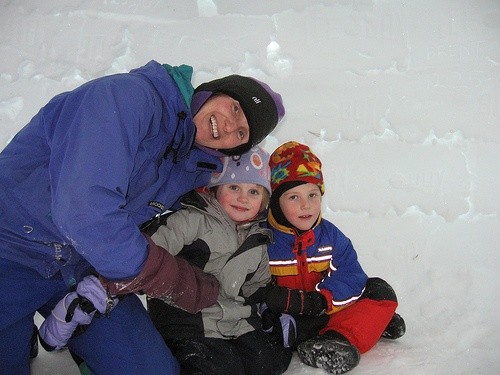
[
  {"left": 269, "top": 141, "right": 325, "bottom": 196},
  {"left": 193, "top": 74, "right": 285, "bottom": 154},
  {"left": 208, "top": 144, "right": 272, "bottom": 198}
]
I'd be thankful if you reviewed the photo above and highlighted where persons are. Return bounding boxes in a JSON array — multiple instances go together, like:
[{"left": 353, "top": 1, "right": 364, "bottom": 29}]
[
  {"left": 37, "top": 143, "right": 297, "bottom": 375},
  {"left": 2, "top": 61, "right": 284, "bottom": 375},
  {"left": 269, "top": 141, "right": 405, "bottom": 375}
]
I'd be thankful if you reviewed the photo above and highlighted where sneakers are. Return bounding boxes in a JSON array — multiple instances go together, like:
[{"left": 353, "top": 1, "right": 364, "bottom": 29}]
[
  {"left": 31, "top": 325, "right": 38, "bottom": 359},
  {"left": 381, "top": 313, "right": 405, "bottom": 340},
  {"left": 297, "top": 335, "right": 359, "bottom": 375},
  {"left": 37, "top": 304, "right": 52, "bottom": 319}
]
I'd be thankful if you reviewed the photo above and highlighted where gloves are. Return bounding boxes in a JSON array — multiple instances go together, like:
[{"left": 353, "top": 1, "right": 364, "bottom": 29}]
[
  {"left": 279, "top": 286, "right": 333, "bottom": 316},
  {"left": 38, "top": 292, "right": 95, "bottom": 353},
  {"left": 101, "top": 233, "right": 220, "bottom": 314},
  {"left": 258, "top": 303, "right": 297, "bottom": 348}
]
